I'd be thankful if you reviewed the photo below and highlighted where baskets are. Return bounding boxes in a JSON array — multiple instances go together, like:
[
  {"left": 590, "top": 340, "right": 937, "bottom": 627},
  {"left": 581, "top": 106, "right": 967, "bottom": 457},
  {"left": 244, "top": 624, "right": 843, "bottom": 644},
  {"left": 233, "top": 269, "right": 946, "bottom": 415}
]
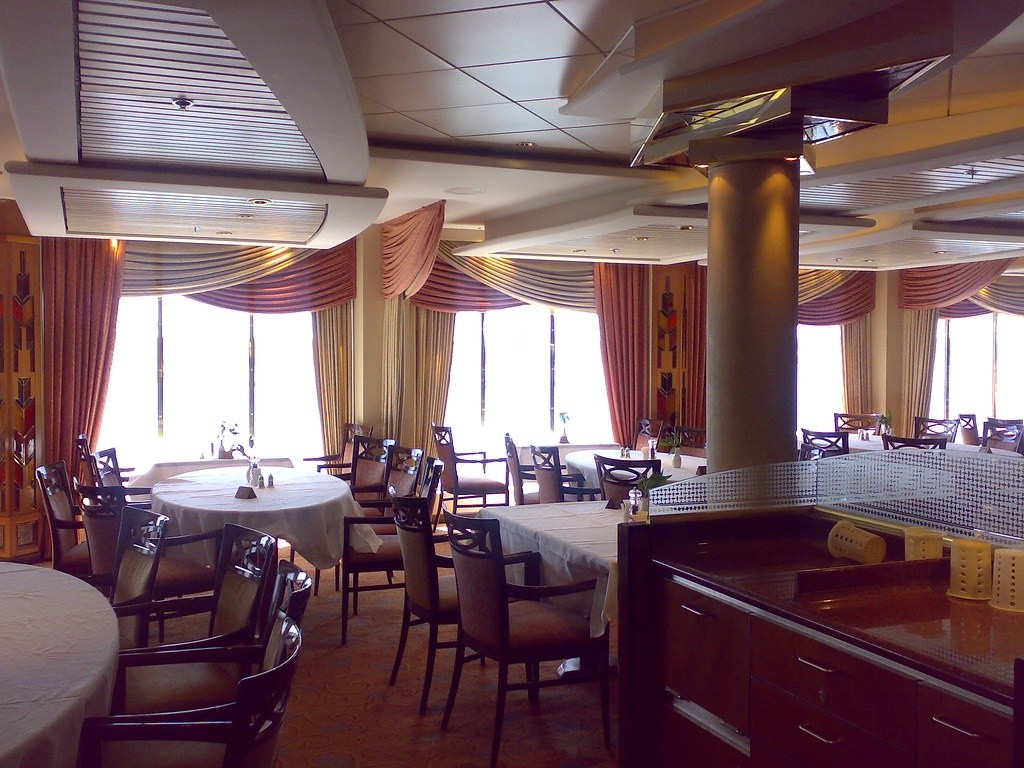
[
  {"left": 944, "top": 538, "right": 992, "bottom": 600},
  {"left": 903, "top": 527, "right": 944, "bottom": 560},
  {"left": 988, "top": 546, "right": 1024, "bottom": 612},
  {"left": 827, "top": 520, "right": 886, "bottom": 565}
]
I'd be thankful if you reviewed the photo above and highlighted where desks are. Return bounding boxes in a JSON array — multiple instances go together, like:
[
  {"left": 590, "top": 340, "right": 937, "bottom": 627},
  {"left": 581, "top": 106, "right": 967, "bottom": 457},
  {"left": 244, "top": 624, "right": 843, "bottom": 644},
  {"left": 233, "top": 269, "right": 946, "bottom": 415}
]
[
  {"left": 565, "top": 448, "right": 706, "bottom": 494},
  {"left": 0, "top": 555, "right": 120, "bottom": 768},
  {"left": 150, "top": 465, "right": 383, "bottom": 570},
  {"left": 127, "top": 455, "right": 294, "bottom": 501},
  {"left": 470, "top": 499, "right": 641, "bottom": 672},
  {"left": 804, "top": 433, "right": 1023, "bottom": 460}
]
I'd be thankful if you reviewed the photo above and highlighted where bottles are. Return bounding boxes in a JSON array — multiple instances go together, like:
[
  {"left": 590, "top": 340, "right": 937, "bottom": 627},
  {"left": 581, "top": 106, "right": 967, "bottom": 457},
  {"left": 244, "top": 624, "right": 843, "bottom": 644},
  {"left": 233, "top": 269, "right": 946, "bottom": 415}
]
[
  {"left": 626, "top": 448, "right": 630, "bottom": 458},
  {"left": 268, "top": 473, "right": 273, "bottom": 488},
  {"left": 860, "top": 432, "right": 864, "bottom": 440},
  {"left": 865, "top": 433, "right": 869, "bottom": 441},
  {"left": 258, "top": 474, "right": 264, "bottom": 488},
  {"left": 672, "top": 448, "right": 681, "bottom": 468},
  {"left": 621, "top": 447, "right": 625, "bottom": 457}
]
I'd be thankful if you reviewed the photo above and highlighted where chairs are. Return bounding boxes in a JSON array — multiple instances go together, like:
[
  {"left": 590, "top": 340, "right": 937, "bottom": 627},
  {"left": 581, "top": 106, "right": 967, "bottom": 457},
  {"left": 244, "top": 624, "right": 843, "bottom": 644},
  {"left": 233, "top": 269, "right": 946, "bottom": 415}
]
[
  {"left": 795, "top": 412, "right": 1024, "bottom": 461},
  {"left": 27, "top": 419, "right": 704, "bottom": 768}
]
